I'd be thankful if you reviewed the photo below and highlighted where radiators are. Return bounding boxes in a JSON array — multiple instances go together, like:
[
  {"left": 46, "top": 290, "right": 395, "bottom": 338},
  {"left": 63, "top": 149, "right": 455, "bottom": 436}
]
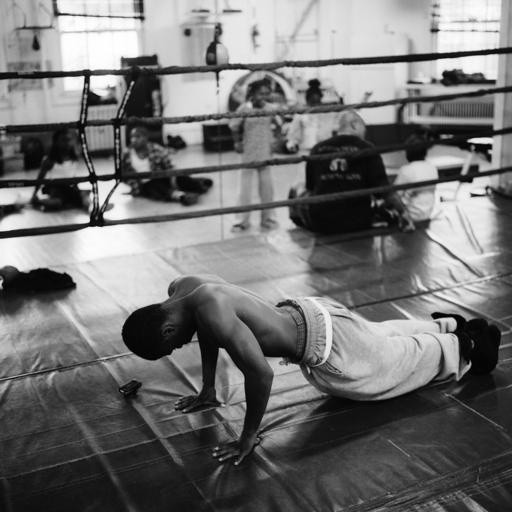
[{"left": 82, "top": 104, "right": 125, "bottom": 152}]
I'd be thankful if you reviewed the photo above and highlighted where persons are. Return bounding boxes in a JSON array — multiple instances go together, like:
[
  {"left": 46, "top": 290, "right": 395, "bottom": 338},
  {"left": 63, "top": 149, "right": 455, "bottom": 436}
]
[
  {"left": 31, "top": 129, "right": 113, "bottom": 216},
  {"left": 229, "top": 80, "right": 283, "bottom": 230},
  {"left": 121, "top": 126, "right": 213, "bottom": 205},
  {"left": 122, "top": 275, "right": 502, "bottom": 467},
  {"left": 286, "top": 79, "right": 417, "bottom": 233},
  {"left": 393, "top": 134, "right": 439, "bottom": 230}
]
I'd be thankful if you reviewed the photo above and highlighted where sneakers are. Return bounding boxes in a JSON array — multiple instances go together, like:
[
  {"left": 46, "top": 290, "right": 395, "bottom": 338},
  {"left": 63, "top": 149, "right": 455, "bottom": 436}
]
[
  {"left": 180, "top": 193, "right": 199, "bottom": 206},
  {"left": 458, "top": 318, "right": 501, "bottom": 374}
]
